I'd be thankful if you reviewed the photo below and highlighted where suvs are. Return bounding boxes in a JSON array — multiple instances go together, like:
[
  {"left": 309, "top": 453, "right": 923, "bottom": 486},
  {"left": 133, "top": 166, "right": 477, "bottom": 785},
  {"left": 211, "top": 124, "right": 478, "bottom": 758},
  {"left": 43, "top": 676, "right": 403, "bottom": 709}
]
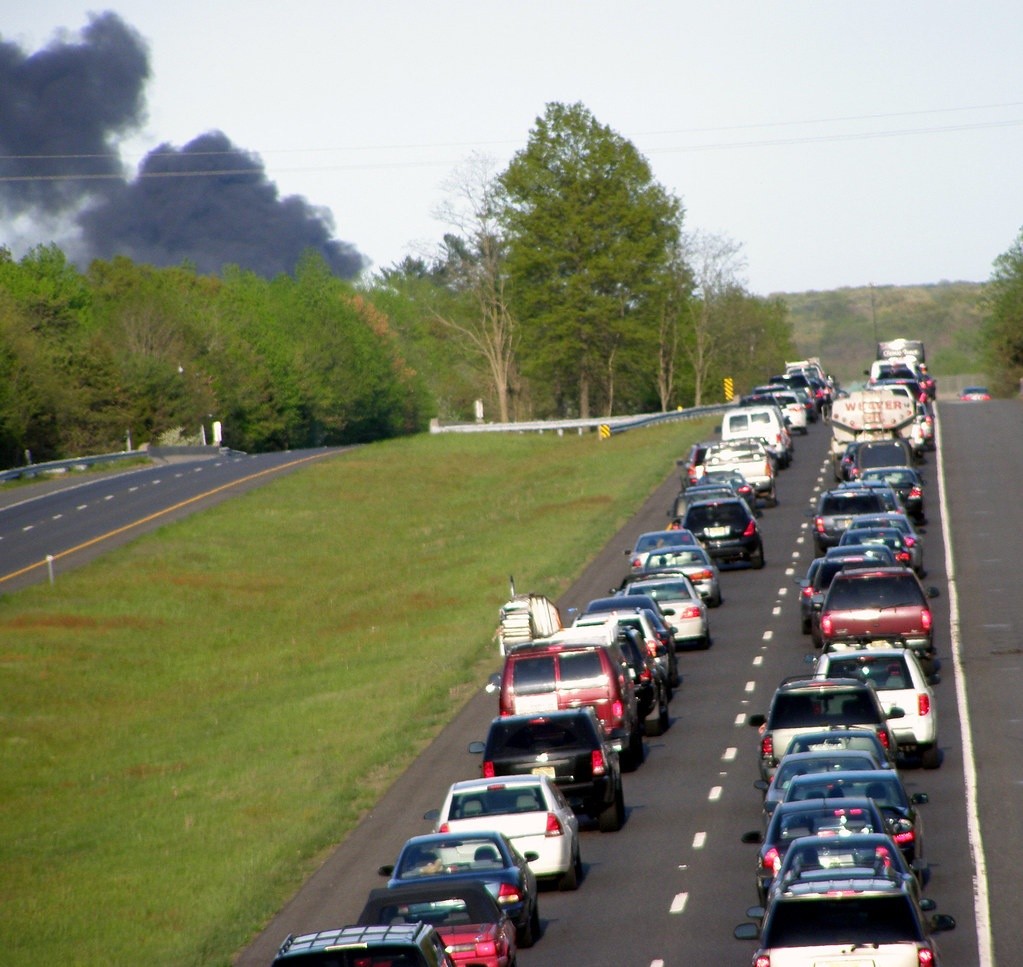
[
  {"left": 268, "top": 921, "right": 455, "bottom": 967},
  {"left": 468, "top": 706, "right": 630, "bottom": 834}
]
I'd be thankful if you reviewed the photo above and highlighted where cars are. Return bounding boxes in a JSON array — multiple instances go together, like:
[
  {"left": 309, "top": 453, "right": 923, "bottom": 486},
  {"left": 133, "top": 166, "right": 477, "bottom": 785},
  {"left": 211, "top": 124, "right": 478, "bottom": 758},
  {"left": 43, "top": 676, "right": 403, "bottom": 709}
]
[
  {"left": 423, "top": 773, "right": 586, "bottom": 892},
  {"left": 956, "top": 387, "right": 991, "bottom": 401},
  {"left": 638, "top": 545, "right": 724, "bottom": 609},
  {"left": 378, "top": 831, "right": 541, "bottom": 950},
  {"left": 609, "top": 568, "right": 704, "bottom": 594},
  {"left": 358, "top": 879, "right": 520, "bottom": 967},
  {"left": 770, "top": 729, "right": 892, "bottom": 775},
  {"left": 622, "top": 578, "right": 712, "bottom": 651},
  {"left": 734, "top": 863, "right": 956, "bottom": 967},
  {"left": 742, "top": 797, "right": 902, "bottom": 909},
  {"left": 667, "top": 338, "right": 937, "bottom": 771},
  {"left": 752, "top": 749, "right": 891, "bottom": 812},
  {"left": 541, "top": 593, "right": 682, "bottom": 739},
  {"left": 785, "top": 766, "right": 929, "bottom": 865},
  {"left": 624, "top": 529, "right": 706, "bottom": 574}
]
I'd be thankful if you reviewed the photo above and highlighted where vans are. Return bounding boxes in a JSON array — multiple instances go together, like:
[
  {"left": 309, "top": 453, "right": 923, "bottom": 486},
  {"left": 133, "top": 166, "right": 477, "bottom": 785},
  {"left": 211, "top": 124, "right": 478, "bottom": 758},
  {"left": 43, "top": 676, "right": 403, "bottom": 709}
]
[{"left": 489, "top": 640, "right": 648, "bottom": 774}]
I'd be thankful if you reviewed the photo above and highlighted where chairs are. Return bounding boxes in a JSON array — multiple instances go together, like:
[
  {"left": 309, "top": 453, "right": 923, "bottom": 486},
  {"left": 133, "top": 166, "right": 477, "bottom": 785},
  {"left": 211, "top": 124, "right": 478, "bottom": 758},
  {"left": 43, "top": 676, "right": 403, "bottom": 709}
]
[
  {"left": 418, "top": 852, "right": 438, "bottom": 868},
  {"left": 865, "top": 782, "right": 886, "bottom": 799},
  {"left": 827, "top": 787, "right": 844, "bottom": 798},
  {"left": 787, "top": 816, "right": 814, "bottom": 836},
  {"left": 473, "top": 846, "right": 499, "bottom": 862},
  {"left": 796, "top": 769, "right": 807, "bottom": 775},
  {"left": 842, "top": 698, "right": 861, "bottom": 716},
  {"left": 805, "top": 791, "right": 825, "bottom": 799},
  {"left": 797, "top": 745, "right": 810, "bottom": 753},
  {"left": 802, "top": 850, "right": 818, "bottom": 864}
]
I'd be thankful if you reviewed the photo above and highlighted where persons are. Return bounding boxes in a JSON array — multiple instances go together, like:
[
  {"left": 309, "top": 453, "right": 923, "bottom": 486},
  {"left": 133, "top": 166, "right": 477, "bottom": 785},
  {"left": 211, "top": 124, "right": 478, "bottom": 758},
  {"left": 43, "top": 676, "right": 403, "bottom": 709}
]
[
  {"left": 865, "top": 782, "right": 889, "bottom": 802},
  {"left": 660, "top": 558, "right": 666, "bottom": 567},
  {"left": 844, "top": 663, "right": 861, "bottom": 679},
  {"left": 648, "top": 539, "right": 656, "bottom": 552},
  {"left": 691, "top": 553, "right": 700, "bottom": 561},
  {"left": 796, "top": 851, "right": 825, "bottom": 878}
]
